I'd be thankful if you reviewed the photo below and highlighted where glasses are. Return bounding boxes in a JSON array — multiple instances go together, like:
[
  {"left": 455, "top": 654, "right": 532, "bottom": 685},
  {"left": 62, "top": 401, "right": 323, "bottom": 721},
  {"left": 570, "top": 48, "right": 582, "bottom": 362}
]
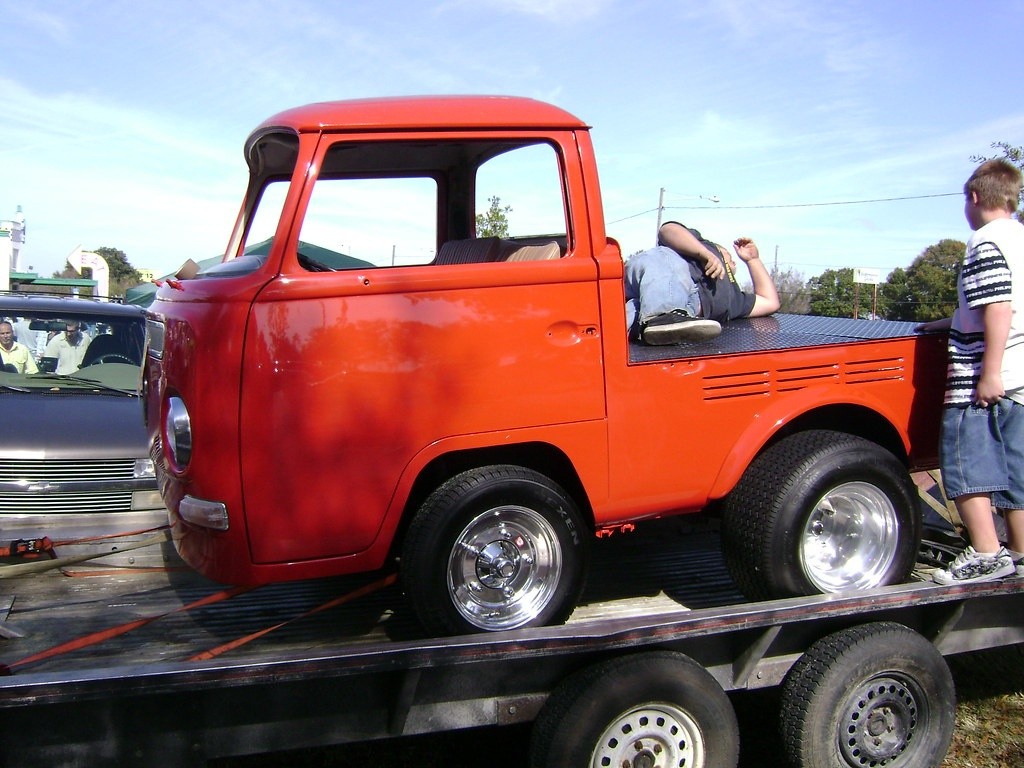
[{"left": 67, "top": 329, "right": 76, "bottom": 333}]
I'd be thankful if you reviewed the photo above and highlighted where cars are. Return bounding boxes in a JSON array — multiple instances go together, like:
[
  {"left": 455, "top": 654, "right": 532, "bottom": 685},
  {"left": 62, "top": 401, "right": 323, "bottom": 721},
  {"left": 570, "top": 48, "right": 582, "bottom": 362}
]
[{"left": 0, "top": 285, "right": 166, "bottom": 521}]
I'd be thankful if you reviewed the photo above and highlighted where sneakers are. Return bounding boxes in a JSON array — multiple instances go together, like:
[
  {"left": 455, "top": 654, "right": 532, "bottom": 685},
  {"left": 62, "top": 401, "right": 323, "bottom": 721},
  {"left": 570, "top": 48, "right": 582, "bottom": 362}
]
[{"left": 933, "top": 541, "right": 1023, "bottom": 584}]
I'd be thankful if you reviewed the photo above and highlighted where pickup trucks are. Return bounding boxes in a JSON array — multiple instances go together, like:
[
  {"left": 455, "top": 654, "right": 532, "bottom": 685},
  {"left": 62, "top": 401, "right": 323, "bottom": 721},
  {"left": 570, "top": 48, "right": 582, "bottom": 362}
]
[{"left": 142, "top": 82, "right": 978, "bottom": 635}]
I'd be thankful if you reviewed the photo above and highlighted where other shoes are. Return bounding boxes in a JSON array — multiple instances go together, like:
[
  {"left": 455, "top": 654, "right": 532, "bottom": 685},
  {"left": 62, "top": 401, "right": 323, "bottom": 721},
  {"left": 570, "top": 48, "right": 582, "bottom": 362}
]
[{"left": 642, "top": 308, "right": 721, "bottom": 347}]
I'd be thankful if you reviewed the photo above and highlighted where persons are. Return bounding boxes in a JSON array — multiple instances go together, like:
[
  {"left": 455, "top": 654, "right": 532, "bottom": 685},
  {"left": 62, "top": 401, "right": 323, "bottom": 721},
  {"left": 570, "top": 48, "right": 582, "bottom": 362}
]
[
  {"left": 914, "top": 160, "right": 1024, "bottom": 584},
  {"left": 0, "top": 316, "right": 112, "bottom": 378},
  {"left": 624, "top": 221, "right": 782, "bottom": 346}
]
